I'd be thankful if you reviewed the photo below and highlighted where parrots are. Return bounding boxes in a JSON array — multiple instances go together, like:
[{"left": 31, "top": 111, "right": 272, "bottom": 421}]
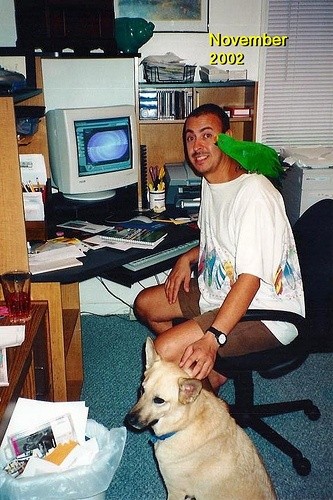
[{"left": 213, "top": 133, "right": 288, "bottom": 192}]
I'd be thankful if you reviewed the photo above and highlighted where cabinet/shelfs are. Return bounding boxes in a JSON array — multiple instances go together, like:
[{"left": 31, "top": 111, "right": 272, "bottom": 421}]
[
  {"left": 0, "top": 51, "right": 85, "bottom": 401},
  {"left": 0, "top": 300, "right": 55, "bottom": 449},
  {"left": 139, "top": 80, "right": 260, "bottom": 210}
]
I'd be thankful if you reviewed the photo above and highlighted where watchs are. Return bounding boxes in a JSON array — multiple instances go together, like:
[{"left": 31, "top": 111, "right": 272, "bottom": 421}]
[{"left": 205, "top": 327, "right": 228, "bottom": 347}]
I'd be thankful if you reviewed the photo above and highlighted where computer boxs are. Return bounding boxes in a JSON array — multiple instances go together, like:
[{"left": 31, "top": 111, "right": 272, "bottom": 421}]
[{"left": 282, "top": 164, "right": 333, "bottom": 226}]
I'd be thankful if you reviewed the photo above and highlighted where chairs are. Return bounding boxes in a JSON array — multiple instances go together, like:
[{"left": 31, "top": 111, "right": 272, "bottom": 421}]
[{"left": 177, "top": 198, "right": 333, "bottom": 477}]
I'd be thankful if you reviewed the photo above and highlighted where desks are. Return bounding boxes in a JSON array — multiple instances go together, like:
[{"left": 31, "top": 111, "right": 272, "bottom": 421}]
[{"left": 30, "top": 184, "right": 200, "bottom": 288}]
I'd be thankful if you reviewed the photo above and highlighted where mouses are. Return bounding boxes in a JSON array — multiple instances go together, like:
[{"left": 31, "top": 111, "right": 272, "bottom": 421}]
[{"left": 129, "top": 215, "right": 154, "bottom": 224}]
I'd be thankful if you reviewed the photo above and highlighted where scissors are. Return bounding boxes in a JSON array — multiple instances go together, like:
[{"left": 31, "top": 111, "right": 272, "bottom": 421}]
[
  {"left": 154, "top": 167, "right": 165, "bottom": 190},
  {"left": 149, "top": 166, "right": 159, "bottom": 182}
]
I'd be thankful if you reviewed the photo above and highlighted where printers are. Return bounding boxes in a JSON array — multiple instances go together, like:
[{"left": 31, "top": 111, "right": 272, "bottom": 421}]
[{"left": 163, "top": 162, "right": 202, "bottom": 209}]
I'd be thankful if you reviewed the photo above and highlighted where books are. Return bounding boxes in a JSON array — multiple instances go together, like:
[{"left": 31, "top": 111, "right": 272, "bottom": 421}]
[
  {"left": 158, "top": 90, "right": 193, "bottom": 120},
  {"left": 197, "top": 90, "right": 200, "bottom": 109},
  {"left": 223, "top": 105, "right": 250, "bottom": 119},
  {"left": 99, "top": 224, "right": 169, "bottom": 246}
]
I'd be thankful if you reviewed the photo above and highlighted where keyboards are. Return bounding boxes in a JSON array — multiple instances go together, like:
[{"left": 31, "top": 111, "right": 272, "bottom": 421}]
[{"left": 122, "top": 238, "right": 200, "bottom": 272}]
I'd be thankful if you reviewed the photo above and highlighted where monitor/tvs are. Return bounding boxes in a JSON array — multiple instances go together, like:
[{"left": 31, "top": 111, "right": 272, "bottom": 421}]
[{"left": 45, "top": 105, "right": 139, "bottom": 200}]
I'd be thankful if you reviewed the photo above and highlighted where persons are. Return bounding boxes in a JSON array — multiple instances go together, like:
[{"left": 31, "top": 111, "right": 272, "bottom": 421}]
[{"left": 134, "top": 104, "right": 306, "bottom": 392}]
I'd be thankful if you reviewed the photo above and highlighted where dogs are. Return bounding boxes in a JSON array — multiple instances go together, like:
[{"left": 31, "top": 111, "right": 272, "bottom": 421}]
[{"left": 123, "top": 336, "right": 279, "bottom": 500}]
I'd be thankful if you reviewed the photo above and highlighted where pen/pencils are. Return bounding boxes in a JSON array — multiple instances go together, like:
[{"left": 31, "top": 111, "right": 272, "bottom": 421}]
[
  {"left": 36, "top": 178, "right": 40, "bottom": 192},
  {"left": 42, "top": 185, "right": 46, "bottom": 204},
  {"left": 46, "top": 177, "right": 51, "bottom": 202},
  {"left": 22, "top": 181, "right": 35, "bottom": 192}
]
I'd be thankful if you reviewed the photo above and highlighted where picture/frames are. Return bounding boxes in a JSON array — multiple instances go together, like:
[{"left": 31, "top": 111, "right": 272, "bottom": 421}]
[{"left": 114, "top": 0, "right": 209, "bottom": 34}]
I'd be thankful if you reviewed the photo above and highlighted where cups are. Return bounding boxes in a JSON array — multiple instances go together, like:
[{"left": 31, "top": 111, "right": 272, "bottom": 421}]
[
  {"left": 146, "top": 190, "right": 168, "bottom": 213},
  {"left": 1, "top": 271, "right": 36, "bottom": 323}
]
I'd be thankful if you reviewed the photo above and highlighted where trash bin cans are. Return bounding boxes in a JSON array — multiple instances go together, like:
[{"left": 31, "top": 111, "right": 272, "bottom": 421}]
[{"left": 0, "top": 419, "right": 110, "bottom": 499}]
